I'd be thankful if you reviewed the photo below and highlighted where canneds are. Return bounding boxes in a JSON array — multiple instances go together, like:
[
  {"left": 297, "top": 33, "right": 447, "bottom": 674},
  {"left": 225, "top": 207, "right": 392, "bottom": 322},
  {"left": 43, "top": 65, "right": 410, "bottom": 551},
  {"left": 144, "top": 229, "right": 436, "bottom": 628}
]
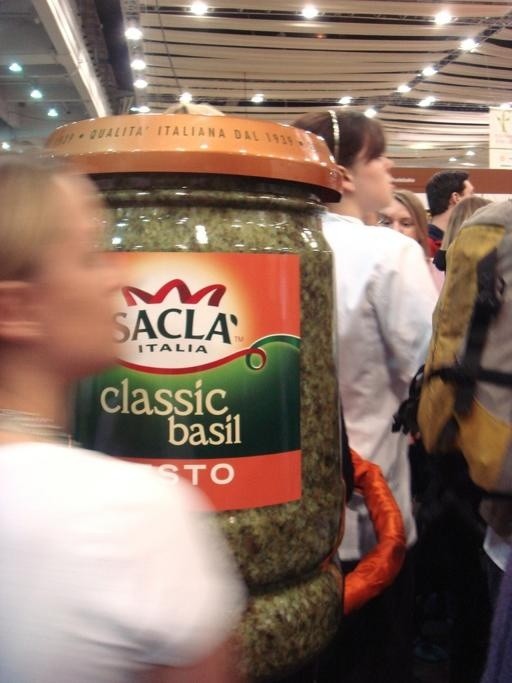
[{"left": 42, "top": 113, "right": 347, "bottom": 679}]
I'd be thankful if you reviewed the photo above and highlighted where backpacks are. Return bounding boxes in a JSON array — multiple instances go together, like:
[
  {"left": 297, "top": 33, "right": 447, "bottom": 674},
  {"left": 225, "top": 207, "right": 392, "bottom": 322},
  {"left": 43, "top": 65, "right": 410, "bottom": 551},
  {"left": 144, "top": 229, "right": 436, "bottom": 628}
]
[{"left": 389, "top": 196, "right": 512, "bottom": 494}]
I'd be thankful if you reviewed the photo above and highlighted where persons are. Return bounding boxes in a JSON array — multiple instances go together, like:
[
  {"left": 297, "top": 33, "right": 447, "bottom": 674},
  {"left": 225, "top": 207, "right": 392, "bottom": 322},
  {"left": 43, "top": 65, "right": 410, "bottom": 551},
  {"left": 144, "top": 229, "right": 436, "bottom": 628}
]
[
  {"left": 0, "top": 153, "right": 253, "bottom": 683},
  {"left": 400, "top": 197, "right": 512, "bottom": 682},
  {"left": 379, "top": 184, "right": 446, "bottom": 299},
  {"left": 289, "top": 104, "right": 446, "bottom": 681},
  {"left": 424, "top": 165, "right": 477, "bottom": 252},
  {"left": 440, "top": 195, "right": 500, "bottom": 254},
  {"left": 424, "top": 208, "right": 433, "bottom": 224}
]
